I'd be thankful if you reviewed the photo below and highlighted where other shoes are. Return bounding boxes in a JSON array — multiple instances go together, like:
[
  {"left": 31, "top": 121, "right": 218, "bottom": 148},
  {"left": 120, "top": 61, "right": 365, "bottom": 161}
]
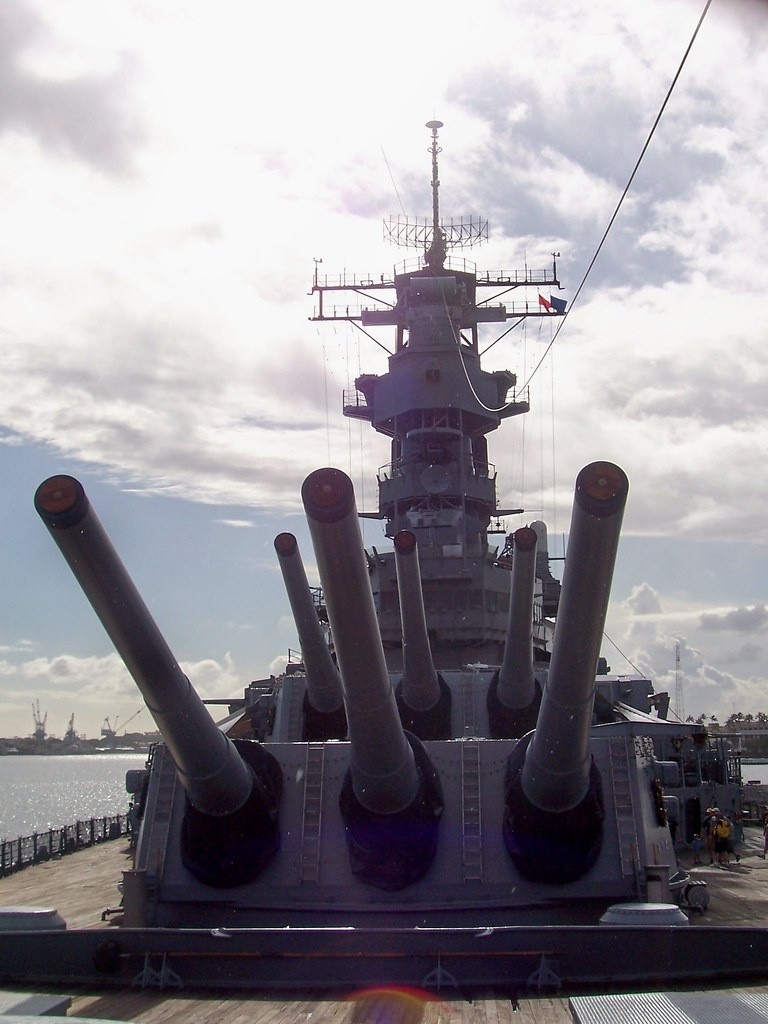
[
  {"left": 710, "top": 861, "right": 731, "bottom": 870},
  {"left": 736, "top": 854, "right": 740, "bottom": 862},
  {"left": 757, "top": 854, "right": 765, "bottom": 859}
]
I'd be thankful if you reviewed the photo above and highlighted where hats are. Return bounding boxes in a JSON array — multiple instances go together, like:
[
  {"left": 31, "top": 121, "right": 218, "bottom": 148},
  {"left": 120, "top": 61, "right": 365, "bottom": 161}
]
[
  {"left": 709, "top": 808, "right": 719, "bottom": 814},
  {"left": 707, "top": 808, "right": 712, "bottom": 813}
]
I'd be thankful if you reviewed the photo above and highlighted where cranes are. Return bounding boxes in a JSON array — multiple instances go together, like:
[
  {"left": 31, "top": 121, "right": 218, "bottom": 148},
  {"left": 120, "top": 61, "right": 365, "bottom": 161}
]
[
  {"left": 30, "top": 696, "right": 49, "bottom": 741},
  {"left": 101, "top": 704, "right": 145, "bottom": 742},
  {"left": 64, "top": 710, "right": 78, "bottom": 740}
]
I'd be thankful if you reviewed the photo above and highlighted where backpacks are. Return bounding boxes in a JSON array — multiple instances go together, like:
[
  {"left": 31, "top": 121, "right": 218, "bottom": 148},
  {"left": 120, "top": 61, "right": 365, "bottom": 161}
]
[{"left": 716, "top": 819, "right": 729, "bottom": 837}]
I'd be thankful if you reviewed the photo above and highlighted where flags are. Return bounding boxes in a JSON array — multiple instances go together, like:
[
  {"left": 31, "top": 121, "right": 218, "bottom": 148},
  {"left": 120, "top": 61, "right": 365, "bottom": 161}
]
[
  {"left": 538, "top": 294, "right": 551, "bottom": 312},
  {"left": 550, "top": 295, "right": 567, "bottom": 313}
]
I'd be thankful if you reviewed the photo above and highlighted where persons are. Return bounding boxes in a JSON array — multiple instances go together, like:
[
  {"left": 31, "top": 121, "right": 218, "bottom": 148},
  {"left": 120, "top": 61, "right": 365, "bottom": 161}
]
[
  {"left": 755, "top": 806, "right": 768, "bottom": 860},
  {"left": 675, "top": 834, "right": 705, "bottom": 864},
  {"left": 700, "top": 808, "right": 744, "bottom": 869}
]
